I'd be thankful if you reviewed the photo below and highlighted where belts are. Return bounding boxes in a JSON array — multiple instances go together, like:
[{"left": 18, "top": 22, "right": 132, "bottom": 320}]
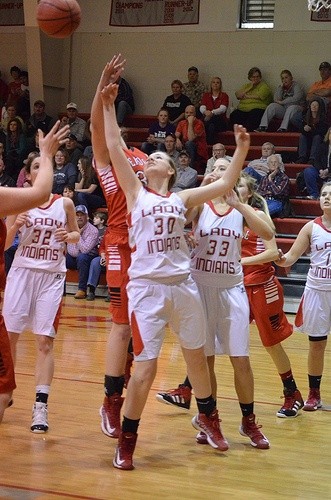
[{"left": 265, "top": 196, "right": 284, "bottom": 203}]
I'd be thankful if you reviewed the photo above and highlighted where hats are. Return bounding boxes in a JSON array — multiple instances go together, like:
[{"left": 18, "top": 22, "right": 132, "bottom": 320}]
[
  {"left": 34, "top": 101, "right": 45, "bottom": 108},
  {"left": 178, "top": 150, "right": 189, "bottom": 158},
  {"left": 65, "top": 133, "right": 77, "bottom": 142},
  {"left": 66, "top": 102, "right": 78, "bottom": 110},
  {"left": 75, "top": 204, "right": 88, "bottom": 216},
  {"left": 165, "top": 132, "right": 177, "bottom": 139},
  {"left": 319, "top": 62, "right": 329, "bottom": 70}
]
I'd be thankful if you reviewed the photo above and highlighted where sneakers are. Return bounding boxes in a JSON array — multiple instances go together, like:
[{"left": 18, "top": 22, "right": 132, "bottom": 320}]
[
  {"left": 30, "top": 402, "right": 48, "bottom": 434},
  {"left": 195, "top": 430, "right": 208, "bottom": 444},
  {"left": 192, "top": 409, "right": 229, "bottom": 451},
  {"left": 155, "top": 384, "right": 192, "bottom": 410},
  {"left": 99, "top": 393, "right": 126, "bottom": 438},
  {"left": 239, "top": 414, "right": 269, "bottom": 449},
  {"left": 276, "top": 389, "right": 304, "bottom": 418},
  {"left": 113, "top": 432, "right": 137, "bottom": 470},
  {"left": 302, "top": 388, "right": 322, "bottom": 411}
]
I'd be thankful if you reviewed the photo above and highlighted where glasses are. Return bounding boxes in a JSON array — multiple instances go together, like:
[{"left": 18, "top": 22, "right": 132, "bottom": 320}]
[
  {"left": 164, "top": 140, "right": 175, "bottom": 143},
  {"left": 212, "top": 149, "right": 225, "bottom": 152}
]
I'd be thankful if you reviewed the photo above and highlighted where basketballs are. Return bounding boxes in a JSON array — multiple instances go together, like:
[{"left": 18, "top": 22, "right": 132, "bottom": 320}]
[{"left": 35, "top": 0, "right": 82, "bottom": 38}]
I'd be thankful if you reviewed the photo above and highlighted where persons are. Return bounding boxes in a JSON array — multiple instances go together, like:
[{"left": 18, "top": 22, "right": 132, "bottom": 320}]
[
  {"left": 175, "top": 104, "right": 208, "bottom": 169},
  {"left": 248, "top": 142, "right": 276, "bottom": 177},
  {"left": 91, "top": 52, "right": 152, "bottom": 438},
  {"left": 51, "top": 149, "right": 77, "bottom": 197},
  {"left": 101, "top": 84, "right": 250, "bottom": 468},
  {"left": 62, "top": 185, "right": 81, "bottom": 207},
  {"left": 0, "top": 51, "right": 331, "bottom": 470},
  {"left": 203, "top": 143, "right": 232, "bottom": 176},
  {"left": 92, "top": 210, "right": 106, "bottom": 237},
  {"left": 0, "top": 118, "right": 71, "bottom": 424},
  {"left": 274, "top": 181, "right": 331, "bottom": 411},
  {"left": 4, "top": 154, "right": 80, "bottom": 432},
  {"left": 200, "top": 76, "right": 229, "bottom": 139},
  {"left": 170, "top": 150, "right": 197, "bottom": 192},
  {"left": 157, "top": 174, "right": 305, "bottom": 418},
  {"left": 258, "top": 154, "right": 289, "bottom": 218},
  {"left": 188, "top": 154, "right": 275, "bottom": 448},
  {"left": 86, "top": 228, "right": 111, "bottom": 302},
  {"left": 63, "top": 204, "right": 99, "bottom": 298},
  {"left": 297, "top": 96, "right": 327, "bottom": 164}
]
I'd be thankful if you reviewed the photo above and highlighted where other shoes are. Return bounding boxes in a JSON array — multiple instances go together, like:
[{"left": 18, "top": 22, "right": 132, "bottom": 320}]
[
  {"left": 294, "top": 191, "right": 317, "bottom": 200},
  {"left": 254, "top": 127, "right": 265, "bottom": 132},
  {"left": 277, "top": 128, "right": 283, "bottom": 132},
  {"left": 87, "top": 292, "right": 95, "bottom": 300},
  {"left": 295, "top": 157, "right": 315, "bottom": 165},
  {"left": 74, "top": 290, "right": 87, "bottom": 298},
  {"left": 105, "top": 296, "right": 110, "bottom": 301}
]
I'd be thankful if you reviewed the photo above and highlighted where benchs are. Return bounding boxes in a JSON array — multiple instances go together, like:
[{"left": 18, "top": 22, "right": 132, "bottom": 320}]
[{"left": 64, "top": 113, "right": 331, "bottom": 314}]
